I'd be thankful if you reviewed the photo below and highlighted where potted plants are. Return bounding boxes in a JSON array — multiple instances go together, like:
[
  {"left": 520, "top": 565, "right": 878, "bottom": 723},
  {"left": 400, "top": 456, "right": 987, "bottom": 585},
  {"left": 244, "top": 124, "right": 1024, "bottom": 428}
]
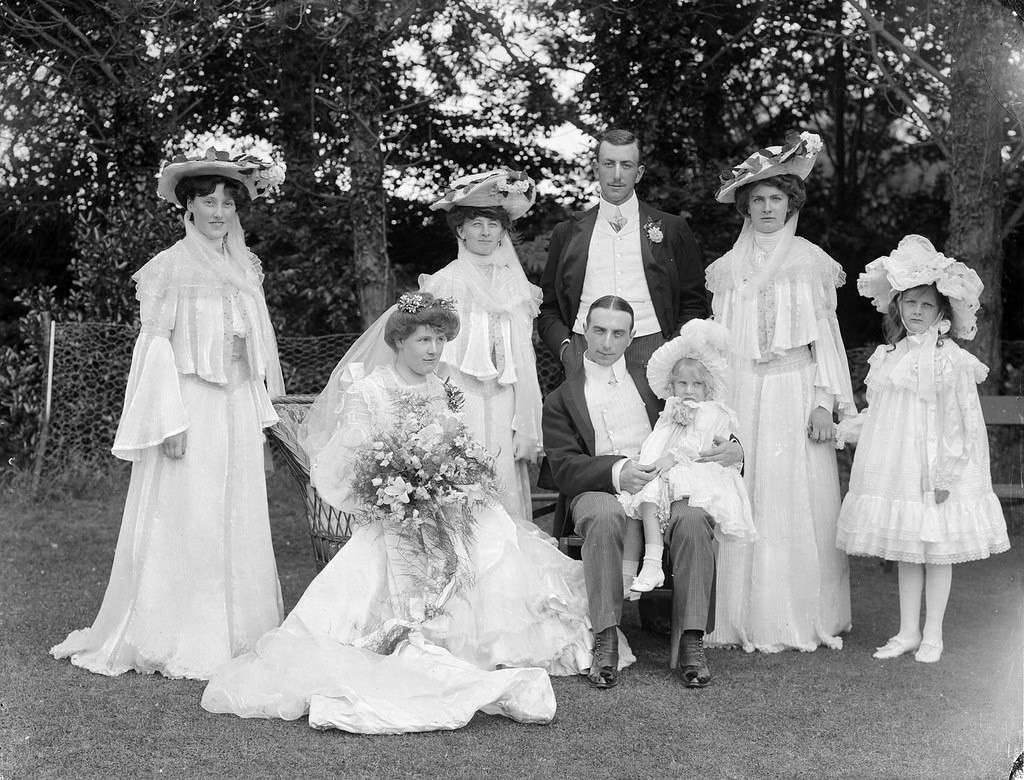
[{"left": 639, "top": 564, "right": 673, "bottom": 638}]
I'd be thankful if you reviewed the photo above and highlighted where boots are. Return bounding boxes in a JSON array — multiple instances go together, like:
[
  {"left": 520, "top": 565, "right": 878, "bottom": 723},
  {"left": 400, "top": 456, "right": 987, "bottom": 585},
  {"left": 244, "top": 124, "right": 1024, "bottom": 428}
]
[
  {"left": 587, "top": 627, "right": 619, "bottom": 688},
  {"left": 679, "top": 635, "right": 711, "bottom": 687}
]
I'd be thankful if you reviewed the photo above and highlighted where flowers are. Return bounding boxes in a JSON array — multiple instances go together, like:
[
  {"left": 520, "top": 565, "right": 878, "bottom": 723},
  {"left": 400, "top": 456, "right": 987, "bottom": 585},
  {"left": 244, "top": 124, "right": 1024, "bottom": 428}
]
[
  {"left": 155, "top": 144, "right": 288, "bottom": 209},
  {"left": 713, "top": 130, "right": 825, "bottom": 199},
  {"left": 430, "top": 164, "right": 535, "bottom": 216},
  {"left": 348, "top": 373, "right": 503, "bottom": 654},
  {"left": 644, "top": 213, "right": 664, "bottom": 246}
]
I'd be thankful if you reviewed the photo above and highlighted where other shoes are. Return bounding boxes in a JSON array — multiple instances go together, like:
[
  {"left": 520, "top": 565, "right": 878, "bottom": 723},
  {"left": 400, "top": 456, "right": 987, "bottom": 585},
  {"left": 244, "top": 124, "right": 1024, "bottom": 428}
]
[
  {"left": 623, "top": 588, "right": 641, "bottom": 601},
  {"left": 630, "top": 561, "right": 665, "bottom": 592},
  {"left": 872, "top": 636, "right": 922, "bottom": 659},
  {"left": 915, "top": 640, "right": 944, "bottom": 664}
]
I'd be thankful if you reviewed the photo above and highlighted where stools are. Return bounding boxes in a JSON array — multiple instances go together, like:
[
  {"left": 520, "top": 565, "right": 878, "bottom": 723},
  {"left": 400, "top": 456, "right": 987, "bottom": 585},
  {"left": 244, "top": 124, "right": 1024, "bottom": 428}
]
[{"left": 560, "top": 534, "right": 683, "bottom": 669}]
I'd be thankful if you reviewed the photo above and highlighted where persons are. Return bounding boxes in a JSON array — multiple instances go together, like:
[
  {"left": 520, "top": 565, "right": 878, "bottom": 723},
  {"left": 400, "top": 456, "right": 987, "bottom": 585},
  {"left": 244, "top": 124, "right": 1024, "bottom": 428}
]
[
  {"left": 415, "top": 167, "right": 543, "bottom": 521},
  {"left": 698, "top": 131, "right": 859, "bottom": 656},
  {"left": 543, "top": 295, "right": 744, "bottom": 688},
  {"left": 200, "top": 289, "right": 637, "bottom": 735},
  {"left": 541, "top": 129, "right": 740, "bottom": 638},
  {"left": 807, "top": 232, "right": 1012, "bottom": 667},
  {"left": 49, "top": 145, "right": 287, "bottom": 680},
  {"left": 619, "top": 316, "right": 759, "bottom": 619}
]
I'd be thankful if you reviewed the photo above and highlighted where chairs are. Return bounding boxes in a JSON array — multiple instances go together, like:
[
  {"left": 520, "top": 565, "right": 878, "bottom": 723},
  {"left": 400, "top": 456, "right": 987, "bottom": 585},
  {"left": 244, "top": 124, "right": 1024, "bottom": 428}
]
[{"left": 267, "top": 392, "right": 356, "bottom": 576}]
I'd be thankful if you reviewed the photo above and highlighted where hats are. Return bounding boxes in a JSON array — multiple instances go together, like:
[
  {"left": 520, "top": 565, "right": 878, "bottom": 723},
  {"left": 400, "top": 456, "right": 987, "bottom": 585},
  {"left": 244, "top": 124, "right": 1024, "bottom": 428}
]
[
  {"left": 714, "top": 131, "right": 822, "bottom": 203},
  {"left": 157, "top": 146, "right": 286, "bottom": 206},
  {"left": 429, "top": 168, "right": 536, "bottom": 221},
  {"left": 857, "top": 234, "right": 984, "bottom": 340},
  {"left": 646, "top": 318, "right": 731, "bottom": 402}
]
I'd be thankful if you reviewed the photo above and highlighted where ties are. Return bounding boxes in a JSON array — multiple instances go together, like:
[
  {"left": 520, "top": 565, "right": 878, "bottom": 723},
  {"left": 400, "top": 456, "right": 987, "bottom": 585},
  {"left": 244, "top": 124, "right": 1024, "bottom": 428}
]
[{"left": 609, "top": 216, "right": 626, "bottom": 232}]
